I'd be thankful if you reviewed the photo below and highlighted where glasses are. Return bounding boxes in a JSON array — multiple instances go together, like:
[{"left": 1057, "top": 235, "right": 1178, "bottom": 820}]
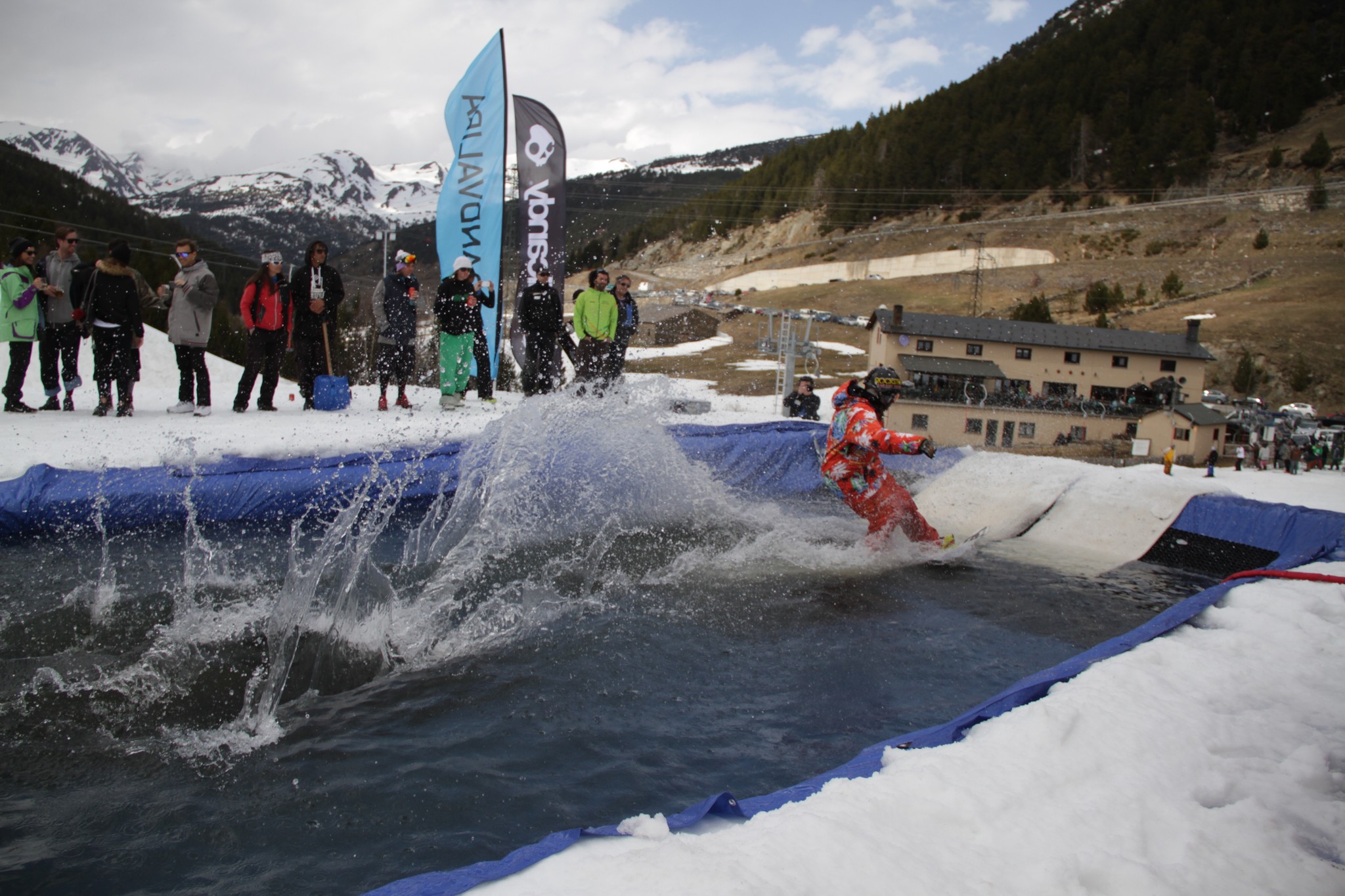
[
  {"left": 316, "top": 249, "right": 327, "bottom": 255},
  {"left": 25, "top": 250, "right": 38, "bottom": 256},
  {"left": 270, "top": 260, "right": 283, "bottom": 265},
  {"left": 176, "top": 251, "right": 193, "bottom": 257},
  {"left": 460, "top": 268, "right": 471, "bottom": 272},
  {"left": 65, "top": 238, "right": 79, "bottom": 244},
  {"left": 395, "top": 255, "right": 416, "bottom": 264},
  {"left": 886, "top": 392, "right": 900, "bottom": 403},
  {"left": 620, "top": 282, "right": 630, "bottom": 288},
  {"left": 538, "top": 272, "right": 550, "bottom": 276}
]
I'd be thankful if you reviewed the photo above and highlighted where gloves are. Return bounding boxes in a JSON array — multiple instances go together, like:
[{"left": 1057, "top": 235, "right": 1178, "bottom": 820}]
[{"left": 919, "top": 439, "right": 936, "bottom": 459}]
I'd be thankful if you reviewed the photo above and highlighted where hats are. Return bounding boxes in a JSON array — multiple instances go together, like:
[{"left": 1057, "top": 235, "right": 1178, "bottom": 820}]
[
  {"left": 394, "top": 249, "right": 417, "bottom": 271},
  {"left": 9, "top": 237, "right": 35, "bottom": 258},
  {"left": 536, "top": 267, "right": 549, "bottom": 273},
  {"left": 453, "top": 256, "right": 472, "bottom": 273}
]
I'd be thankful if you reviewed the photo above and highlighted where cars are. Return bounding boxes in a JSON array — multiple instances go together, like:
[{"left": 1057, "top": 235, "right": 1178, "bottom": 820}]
[
  {"left": 1201, "top": 388, "right": 1228, "bottom": 405},
  {"left": 1320, "top": 410, "right": 1345, "bottom": 430},
  {"left": 1231, "top": 396, "right": 1268, "bottom": 412},
  {"left": 1278, "top": 402, "right": 1318, "bottom": 420},
  {"left": 633, "top": 288, "right": 871, "bottom": 328}
]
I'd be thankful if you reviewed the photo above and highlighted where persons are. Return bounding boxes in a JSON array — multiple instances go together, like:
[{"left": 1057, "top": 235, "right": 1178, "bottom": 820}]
[
  {"left": 157, "top": 239, "right": 219, "bottom": 417},
  {"left": 606, "top": 275, "right": 639, "bottom": 385},
  {"left": 573, "top": 269, "right": 618, "bottom": 398},
  {"left": 372, "top": 247, "right": 425, "bottom": 411},
  {"left": 518, "top": 267, "right": 564, "bottom": 397},
  {"left": 937, "top": 375, "right": 1136, "bottom": 416},
  {"left": 1250, "top": 431, "right": 1340, "bottom": 474},
  {"left": 434, "top": 255, "right": 477, "bottom": 410},
  {"left": 34, "top": 227, "right": 89, "bottom": 411},
  {"left": 460, "top": 268, "right": 496, "bottom": 403},
  {"left": 233, "top": 251, "right": 293, "bottom": 413},
  {"left": 1163, "top": 445, "right": 1176, "bottom": 476},
  {"left": 783, "top": 376, "right": 820, "bottom": 421},
  {"left": 70, "top": 238, "right": 171, "bottom": 417},
  {"left": 0, "top": 237, "right": 42, "bottom": 413},
  {"left": 1053, "top": 432, "right": 1072, "bottom": 446},
  {"left": 821, "top": 367, "right": 956, "bottom": 550},
  {"left": 291, "top": 241, "right": 345, "bottom": 410},
  {"left": 1206, "top": 447, "right": 1218, "bottom": 477},
  {"left": 1234, "top": 444, "right": 1245, "bottom": 471}
]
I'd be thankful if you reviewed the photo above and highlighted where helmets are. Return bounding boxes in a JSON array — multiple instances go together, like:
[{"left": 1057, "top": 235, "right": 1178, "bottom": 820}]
[{"left": 863, "top": 366, "right": 902, "bottom": 393}]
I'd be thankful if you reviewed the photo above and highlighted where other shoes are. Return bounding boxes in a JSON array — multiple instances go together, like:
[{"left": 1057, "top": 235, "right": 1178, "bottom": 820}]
[
  {"left": 2, "top": 398, "right": 315, "bottom": 416},
  {"left": 942, "top": 534, "right": 955, "bottom": 549},
  {"left": 453, "top": 393, "right": 465, "bottom": 406},
  {"left": 460, "top": 395, "right": 466, "bottom": 399},
  {"left": 259, "top": 406, "right": 276, "bottom": 411},
  {"left": 439, "top": 395, "right": 455, "bottom": 410},
  {"left": 481, "top": 397, "right": 496, "bottom": 403}
]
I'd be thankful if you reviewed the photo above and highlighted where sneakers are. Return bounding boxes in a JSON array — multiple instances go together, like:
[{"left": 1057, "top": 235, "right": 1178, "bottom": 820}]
[
  {"left": 379, "top": 396, "right": 388, "bottom": 411},
  {"left": 396, "top": 395, "right": 411, "bottom": 409}
]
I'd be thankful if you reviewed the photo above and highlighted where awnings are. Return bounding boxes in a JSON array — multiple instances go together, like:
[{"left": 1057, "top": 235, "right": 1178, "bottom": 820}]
[
  {"left": 1129, "top": 383, "right": 1154, "bottom": 392},
  {"left": 1151, "top": 377, "right": 1182, "bottom": 389},
  {"left": 898, "top": 354, "right": 1006, "bottom": 407}
]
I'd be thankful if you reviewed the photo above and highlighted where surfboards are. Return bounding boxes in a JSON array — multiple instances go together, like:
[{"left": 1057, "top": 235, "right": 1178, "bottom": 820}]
[{"left": 700, "top": 525, "right": 989, "bottom": 578}]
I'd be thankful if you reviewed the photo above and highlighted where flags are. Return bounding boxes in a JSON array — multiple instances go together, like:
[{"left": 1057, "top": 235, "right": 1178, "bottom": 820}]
[
  {"left": 436, "top": 28, "right": 507, "bottom": 379},
  {"left": 512, "top": 95, "right": 564, "bottom": 301}
]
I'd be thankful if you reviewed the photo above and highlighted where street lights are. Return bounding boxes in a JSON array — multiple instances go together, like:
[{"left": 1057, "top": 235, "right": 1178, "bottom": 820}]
[{"left": 374, "top": 229, "right": 396, "bottom": 278}]
